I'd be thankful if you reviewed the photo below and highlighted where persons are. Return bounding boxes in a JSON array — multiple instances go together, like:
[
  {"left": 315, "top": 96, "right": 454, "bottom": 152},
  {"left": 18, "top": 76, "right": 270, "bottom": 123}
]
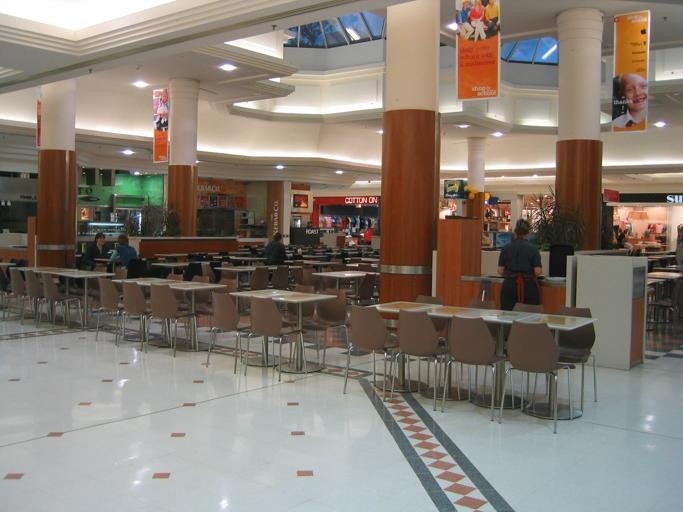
[
  {"left": 498, "top": 219, "right": 542, "bottom": 310},
  {"left": 262, "top": 232, "right": 289, "bottom": 265},
  {"left": 457, "top": 1, "right": 498, "bottom": 41},
  {"left": 617, "top": 229, "right": 633, "bottom": 255},
  {"left": 156, "top": 90, "right": 168, "bottom": 126},
  {"left": 110, "top": 235, "right": 137, "bottom": 272},
  {"left": 81, "top": 233, "right": 111, "bottom": 270},
  {"left": 612, "top": 73, "right": 648, "bottom": 130}
]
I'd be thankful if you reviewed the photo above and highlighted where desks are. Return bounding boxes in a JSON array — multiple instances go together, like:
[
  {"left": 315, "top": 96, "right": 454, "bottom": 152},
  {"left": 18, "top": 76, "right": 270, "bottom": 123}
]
[{"left": 647, "top": 272, "right": 683, "bottom": 329}]
